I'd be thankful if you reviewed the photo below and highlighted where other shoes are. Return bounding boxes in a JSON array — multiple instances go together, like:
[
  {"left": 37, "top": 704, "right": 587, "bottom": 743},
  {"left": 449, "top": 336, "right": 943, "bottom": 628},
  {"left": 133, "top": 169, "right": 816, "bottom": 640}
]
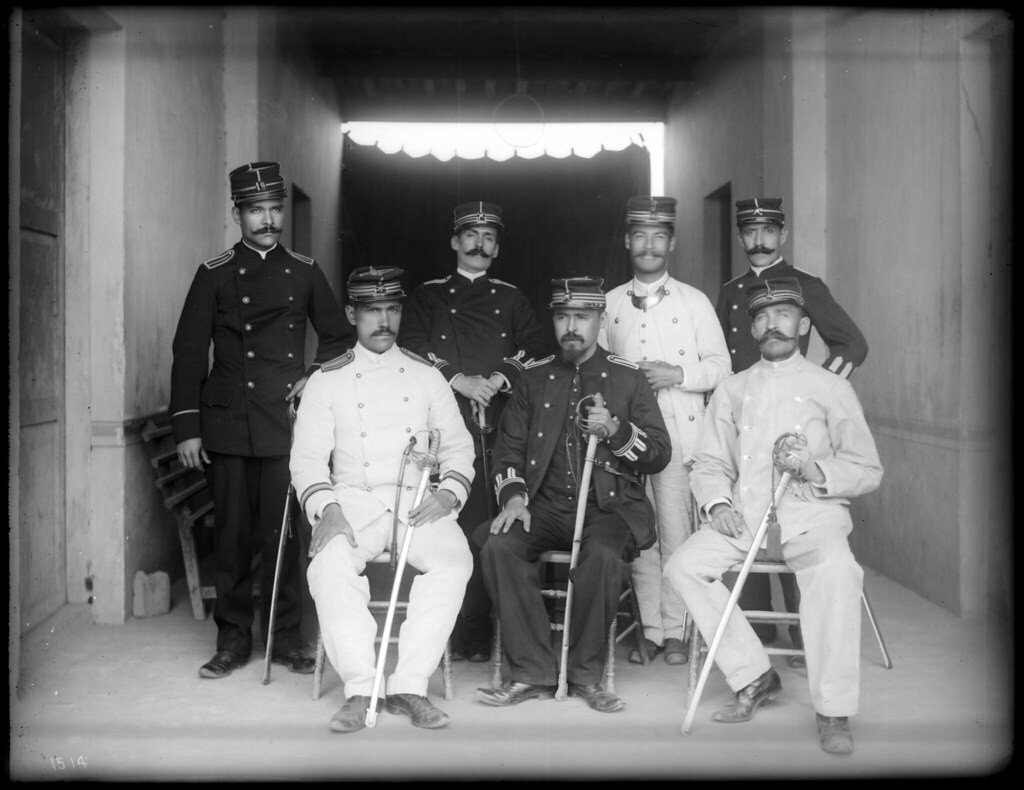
[
  {"left": 787, "top": 633, "right": 804, "bottom": 667},
  {"left": 664, "top": 637, "right": 689, "bottom": 664},
  {"left": 629, "top": 639, "right": 664, "bottom": 664},
  {"left": 452, "top": 646, "right": 476, "bottom": 660},
  {"left": 755, "top": 621, "right": 777, "bottom": 644},
  {"left": 471, "top": 651, "right": 492, "bottom": 661}
]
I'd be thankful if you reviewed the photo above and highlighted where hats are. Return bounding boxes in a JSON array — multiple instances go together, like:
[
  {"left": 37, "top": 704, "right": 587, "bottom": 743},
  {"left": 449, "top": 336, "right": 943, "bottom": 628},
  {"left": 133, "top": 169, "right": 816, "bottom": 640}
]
[
  {"left": 626, "top": 196, "right": 677, "bottom": 227},
  {"left": 745, "top": 276, "right": 807, "bottom": 317},
  {"left": 453, "top": 201, "right": 505, "bottom": 235},
  {"left": 346, "top": 266, "right": 407, "bottom": 304},
  {"left": 548, "top": 276, "right": 606, "bottom": 310},
  {"left": 228, "top": 162, "right": 287, "bottom": 206},
  {"left": 736, "top": 198, "right": 784, "bottom": 229}
]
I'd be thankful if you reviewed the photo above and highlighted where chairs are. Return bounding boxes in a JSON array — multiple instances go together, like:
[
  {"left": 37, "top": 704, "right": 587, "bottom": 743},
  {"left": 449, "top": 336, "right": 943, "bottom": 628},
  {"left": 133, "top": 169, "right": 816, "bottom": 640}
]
[{"left": 124, "top": 412, "right": 294, "bottom": 619}]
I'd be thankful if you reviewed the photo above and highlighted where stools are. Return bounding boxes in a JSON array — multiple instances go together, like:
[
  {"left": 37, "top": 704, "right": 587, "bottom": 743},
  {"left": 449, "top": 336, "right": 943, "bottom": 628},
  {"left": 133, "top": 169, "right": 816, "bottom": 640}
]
[
  {"left": 487, "top": 547, "right": 651, "bottom": 701},
  {"left": 684, "top": 559, "right": 891, "bottom": 711},
  {"left": 308, "top": 551, "right": 451, "bottom": 702}
]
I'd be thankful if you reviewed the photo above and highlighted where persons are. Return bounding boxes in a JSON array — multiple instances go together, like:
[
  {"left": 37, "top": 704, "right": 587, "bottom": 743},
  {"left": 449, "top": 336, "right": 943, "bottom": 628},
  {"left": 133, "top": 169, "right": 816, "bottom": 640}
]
[
  {"left": 289, "top": 269, "right": 477, "bottom": 730},
  {"left": 716, "top": 198, "right": 869, "bottom": 666},
  {"left": 666, "top": 276, "right": 883, "bottom": 754},
  {"left": 478, "top": 278, "right": 673, "bottom": 712},
  {"left": 597, "top": 197, "right": 732, "bottom": 665},
  {"left": 172, "top": 161, "right": 340, "bottom": 676},
  {"left": 406, "top": 202, "right": 545, "bottom": 662}
]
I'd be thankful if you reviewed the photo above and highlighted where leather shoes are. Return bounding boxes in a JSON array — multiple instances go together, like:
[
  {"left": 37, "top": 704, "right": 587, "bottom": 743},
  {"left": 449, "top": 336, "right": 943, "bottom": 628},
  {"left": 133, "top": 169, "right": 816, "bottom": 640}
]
[
  {"left": 386, "top": 693, "right": 451, "bottom": 729},
  {"left": 474, "top": 679, "right": 556, "bottom": 706},
  {"left": 272, "top": 646, "right": 316, "bottom": 675},
  {"left": 567, "top": 680, "right": 623, "bottom": 712},
  {"left": 200, "top": 649, "right": 249, "bottom": 678},
  {"left": 815, "top": 712, "right": 854, "bottom": 752},
  {"left": 331, "top": 695, "right": 384, "bottom": 731},
  {"left": 713, "top": 667, "right": 783, "bottom": 723}
]
